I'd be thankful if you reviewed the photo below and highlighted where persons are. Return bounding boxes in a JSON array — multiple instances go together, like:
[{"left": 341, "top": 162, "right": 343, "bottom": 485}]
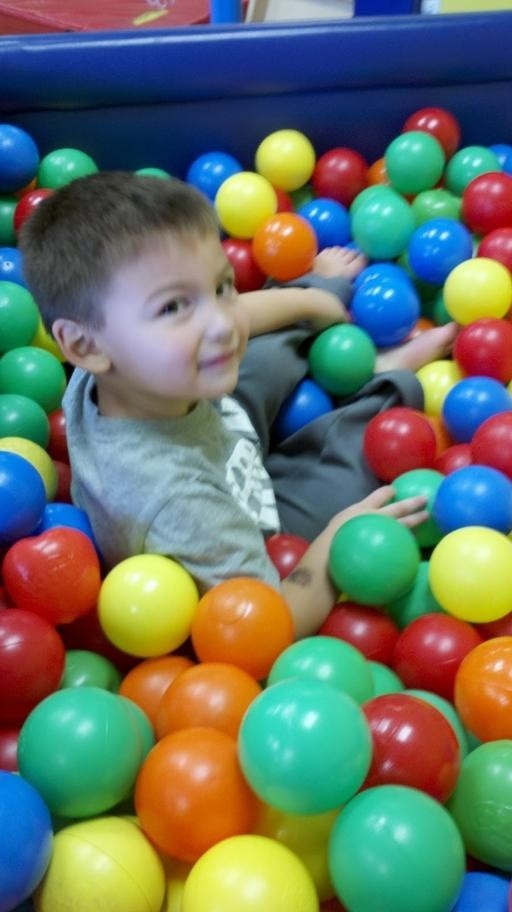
[{"left": 25, "top": 170, "right": 461, "bottom": 637}]
